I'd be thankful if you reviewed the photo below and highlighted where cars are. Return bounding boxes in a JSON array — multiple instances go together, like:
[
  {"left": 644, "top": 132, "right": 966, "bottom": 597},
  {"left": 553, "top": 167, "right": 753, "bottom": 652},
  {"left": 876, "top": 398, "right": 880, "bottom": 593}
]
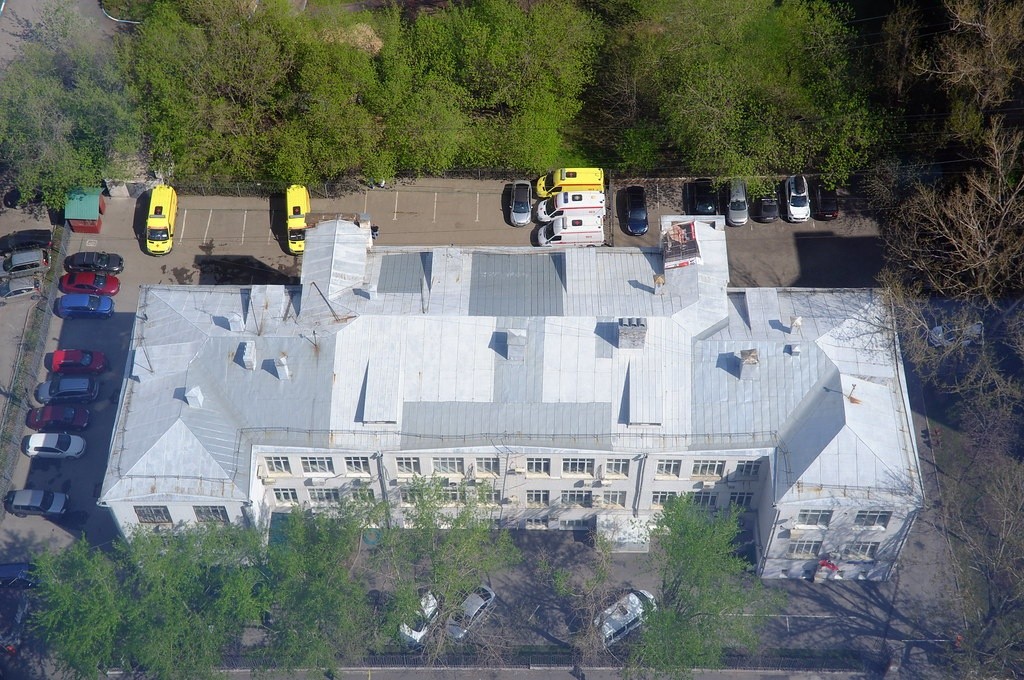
[
  {"left": 929, "top": 322, "right": 984, "bottom": 349},
  {"left": 60, "top": 273, "right": 120, "bottom": 296},
  {"left": 38, "top": 378, "right": 99, "bottom": 404},
  {"left": 443, "top": 585, "right": 496, "bottom": 643},
  {"left": 27, "top": 405, "right": 90, "bottom": 430},
  {"left": 8, "top": 489, "right": 70, "bottom": 517},
  {"left": 759, "top": 197, "right": 778, "bottom": 223},
  {"left": 55, "top": 293, "right": 114, "bottom": 319},
  {"left": 64, "top": 252, "right": 124, "bottom": 276},
  {"left": 626, "top": 186, "right": 649, "bottom": 236},
  {"left": 26, "top": 432, "right": 86, "bottom": 459},
  {"left": 52, "top": 350, "right": 106, "bottom": 376},
  {"left": 509, "top": 180, "right": 534, "bottom": 228},
  {"left": 692, "top": 179, "right": 716, "bottom": 215},
  {"left": 397, "top": 589, "right": 444, "bottom": 648},
  {"left": 814, "top": 176, "right": 838, "bottom": 219}
]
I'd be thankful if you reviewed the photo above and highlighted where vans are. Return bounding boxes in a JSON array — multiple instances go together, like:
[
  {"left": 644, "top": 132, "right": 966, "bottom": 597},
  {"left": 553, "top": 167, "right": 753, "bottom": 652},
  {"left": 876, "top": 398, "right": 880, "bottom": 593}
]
[
  {"left": 725, "top": 178, "right": 749, "bottom": 226},
  {"left": 0, "top": 276, "right": 41, "bottom": 307},
  {"left": 593, "top": 589, "right": 658, "bottom": 652},
  {"left": 0, "top": 249, "right": 51, "bottom": 282},
  {"left": 0, "top": 229, "right": 52, "bottom": 256}
]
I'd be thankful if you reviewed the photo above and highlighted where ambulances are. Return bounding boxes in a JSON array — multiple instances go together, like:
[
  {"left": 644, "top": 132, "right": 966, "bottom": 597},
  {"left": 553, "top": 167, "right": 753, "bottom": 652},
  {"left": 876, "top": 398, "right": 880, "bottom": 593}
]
[
  {"left": 536, "top": 191, "right": 606, "bottom": 221},
  {"left": 286, "top": 184, "right": 311, "bottom": 255},
  {"left": 537, "top": 168, "right": 604, "bottom": 198},
  {"left": 537, "top": 216, "right": 605, "bottom": 248},
  {"left": 144, "top": 185, "right": 178, "bottom": 256}
]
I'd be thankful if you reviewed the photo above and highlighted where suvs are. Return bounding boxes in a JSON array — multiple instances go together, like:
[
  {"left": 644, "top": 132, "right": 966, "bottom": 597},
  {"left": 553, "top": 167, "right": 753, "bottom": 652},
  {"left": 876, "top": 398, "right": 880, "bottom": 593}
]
[{"left": 785, "top": 176, "right": 811, "bottom": 222}]
